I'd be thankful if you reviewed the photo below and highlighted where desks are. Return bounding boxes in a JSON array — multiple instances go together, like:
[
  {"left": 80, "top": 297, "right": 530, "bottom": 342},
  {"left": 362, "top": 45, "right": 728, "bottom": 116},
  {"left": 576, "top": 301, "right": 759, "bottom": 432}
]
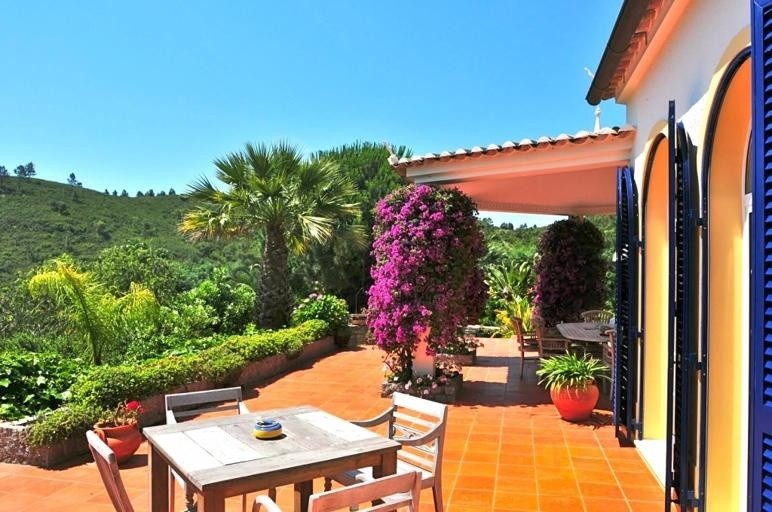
[
  {"left": 132, "top": 402, "right": 405, "bottom": 512},
  {"left": 555, "top": 323, "right": 616, "bottom": 385}
]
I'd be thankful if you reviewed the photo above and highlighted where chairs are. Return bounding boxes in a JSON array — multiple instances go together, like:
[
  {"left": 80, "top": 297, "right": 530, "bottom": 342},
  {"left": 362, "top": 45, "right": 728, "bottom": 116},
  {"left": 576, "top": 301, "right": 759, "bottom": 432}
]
[
  {"left": 252, "top": 467, "right": 425, "bottom": 512},
  {"left": 85, "top": 429, "right": 135, "bottom": 512},
  {"left": 580, "top": 309, "right": 613, "bottom": 323},
  {"left": 164, "top": 386, "right": 255, "bottom": 512},
  {"left": 601, "top": 329, "right": 616, "bottom": 401},
  {"left": 533, "top": 325, "right": 575, "bottom": 384},
  {"left": 510, "top": 316, "right": 548, "bottom": 377},
  {"left": 322, "top": 388, "right": 448, "bottom": 512}
]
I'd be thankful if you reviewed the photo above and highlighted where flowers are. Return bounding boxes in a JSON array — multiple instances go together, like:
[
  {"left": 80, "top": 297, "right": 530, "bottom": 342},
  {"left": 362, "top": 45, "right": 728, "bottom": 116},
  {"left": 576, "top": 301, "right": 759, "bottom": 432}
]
[{"left": 109, "top": 398, "right": 146, "bottom": 428}]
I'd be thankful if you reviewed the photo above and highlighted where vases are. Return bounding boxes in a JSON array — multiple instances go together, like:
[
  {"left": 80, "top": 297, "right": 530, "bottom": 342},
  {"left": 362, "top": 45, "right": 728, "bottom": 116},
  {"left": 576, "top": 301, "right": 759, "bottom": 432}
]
[{"left": 92, "top": 418, "right": 145, "bottom": 467}]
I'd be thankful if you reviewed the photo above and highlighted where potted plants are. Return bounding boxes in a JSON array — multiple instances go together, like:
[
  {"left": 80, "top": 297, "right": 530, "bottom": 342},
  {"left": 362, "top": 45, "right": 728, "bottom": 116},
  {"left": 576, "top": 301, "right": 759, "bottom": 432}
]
[{"left": 532, "top": 348, "right": 614, "bottom": 423}]
